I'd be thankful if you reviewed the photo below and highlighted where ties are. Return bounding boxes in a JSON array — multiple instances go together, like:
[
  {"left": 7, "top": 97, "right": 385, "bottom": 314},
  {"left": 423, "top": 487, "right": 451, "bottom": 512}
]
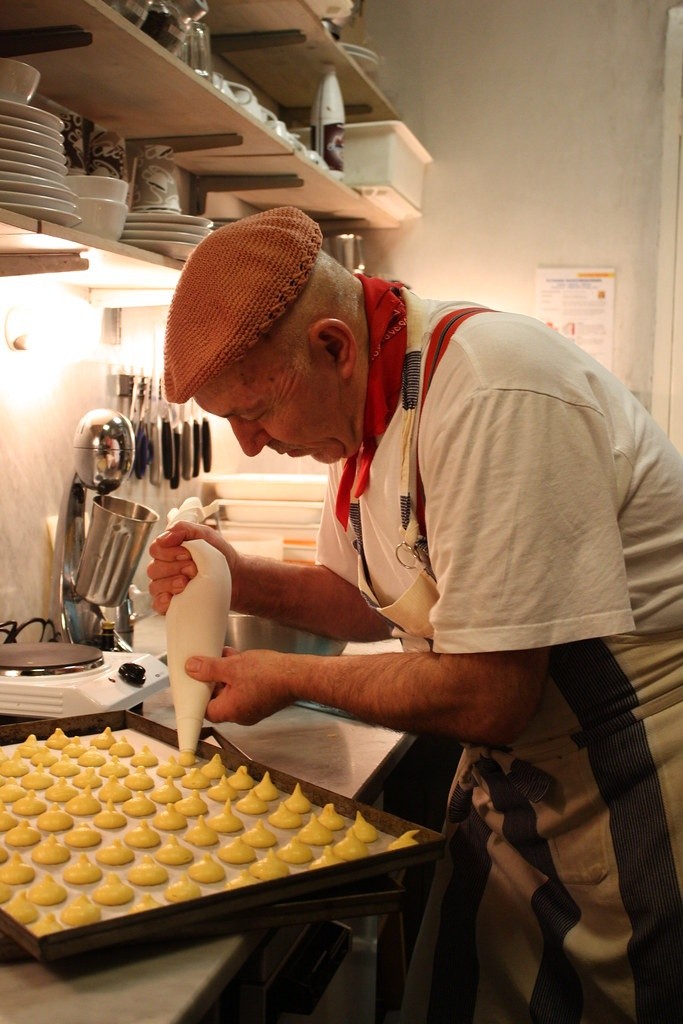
[{"left": 336, "top": 273, "right": 407, "bottom": 530}]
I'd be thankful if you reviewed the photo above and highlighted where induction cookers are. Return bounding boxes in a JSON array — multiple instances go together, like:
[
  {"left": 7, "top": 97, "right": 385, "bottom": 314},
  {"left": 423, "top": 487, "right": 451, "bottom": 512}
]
[{"left": 0, "top": 641, "right": 169, "bottom": 718}]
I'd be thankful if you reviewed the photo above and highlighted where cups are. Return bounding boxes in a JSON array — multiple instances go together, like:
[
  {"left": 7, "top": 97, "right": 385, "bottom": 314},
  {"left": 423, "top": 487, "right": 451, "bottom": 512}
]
[
  {"left": 175, "top": 22, "right": 212, "bottom": 78},
  {"left": 46, "top": 108, "right": 182, "bottom": 214},
  {"left": 75, "top": 493, "right": 158, "bottom": 608}
]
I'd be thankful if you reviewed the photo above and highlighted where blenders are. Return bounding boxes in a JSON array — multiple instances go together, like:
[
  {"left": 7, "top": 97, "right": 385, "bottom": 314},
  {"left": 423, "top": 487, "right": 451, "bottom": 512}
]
[{"left": 47, "top": 408, "right": 159, "bottom": 653}]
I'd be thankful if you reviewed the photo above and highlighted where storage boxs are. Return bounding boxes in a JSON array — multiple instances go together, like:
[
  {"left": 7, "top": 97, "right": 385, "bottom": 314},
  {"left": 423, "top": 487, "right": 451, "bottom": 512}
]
[{"left": 283, "top": 118, "right": 436, "bottom": 226}]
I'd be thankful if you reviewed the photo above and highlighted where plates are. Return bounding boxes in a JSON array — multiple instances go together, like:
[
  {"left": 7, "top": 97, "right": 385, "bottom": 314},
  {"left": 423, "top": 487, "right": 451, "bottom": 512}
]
[
  {"left": 337, "top": 38, "right": 380, "bottom": 72},
  {"left": 0, "top": 97, "right": 83, "bottom": 229},
  {"left": 118, "top": 212, "right": 214, "bottom": 260}
]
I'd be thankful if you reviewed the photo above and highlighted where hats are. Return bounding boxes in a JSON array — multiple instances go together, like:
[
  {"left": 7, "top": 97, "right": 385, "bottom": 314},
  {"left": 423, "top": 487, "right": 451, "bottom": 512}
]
[{"left": 164, "top": 205, "right": 323, "bottom": 405}]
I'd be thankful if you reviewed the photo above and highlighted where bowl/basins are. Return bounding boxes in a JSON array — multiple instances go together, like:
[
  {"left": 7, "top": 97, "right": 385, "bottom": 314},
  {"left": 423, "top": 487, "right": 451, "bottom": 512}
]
[
  {"left": 0, "top": 55, "right": 42, "bottom": 105},
  {"left": 62, "top": 175, "right": 130, "bottom": 243},
  {"left": 224, "top": 609, "right": 348, "bottom": 658},
  {"left": 212, "top": 73, "right": 344, "bottom": 179}
]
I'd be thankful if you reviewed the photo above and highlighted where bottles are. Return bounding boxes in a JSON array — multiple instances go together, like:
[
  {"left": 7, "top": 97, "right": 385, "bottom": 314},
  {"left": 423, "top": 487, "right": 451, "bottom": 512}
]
[
  {"left": 311, "top": 62, "right": 343, "bottom": 172},
  {"left": 102, "top": 621, "right": 115, "bottom": 650}
]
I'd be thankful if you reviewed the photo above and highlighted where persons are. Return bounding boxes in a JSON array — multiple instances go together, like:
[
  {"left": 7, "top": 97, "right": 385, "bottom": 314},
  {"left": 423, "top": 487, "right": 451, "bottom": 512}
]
[
  {"left": 145, "top": 206, "right": 683, "bottom": 1024},
  {"left": 104, "top": 434, "right": 120, "bottom": 464}
]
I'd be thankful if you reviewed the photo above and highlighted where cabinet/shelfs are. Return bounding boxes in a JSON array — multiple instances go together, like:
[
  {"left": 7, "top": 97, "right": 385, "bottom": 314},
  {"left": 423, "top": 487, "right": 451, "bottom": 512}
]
[{"left": 0, "top": 0, "right": 418, "bottom": 308}]
